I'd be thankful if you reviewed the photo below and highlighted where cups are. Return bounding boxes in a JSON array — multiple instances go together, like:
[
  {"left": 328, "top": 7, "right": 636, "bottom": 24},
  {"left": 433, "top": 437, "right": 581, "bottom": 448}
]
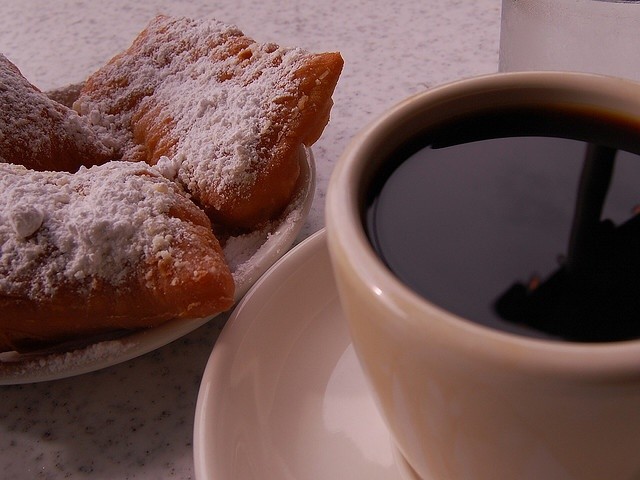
[{"left": 324, "top": 70, "right": 640, "bottom": 477}]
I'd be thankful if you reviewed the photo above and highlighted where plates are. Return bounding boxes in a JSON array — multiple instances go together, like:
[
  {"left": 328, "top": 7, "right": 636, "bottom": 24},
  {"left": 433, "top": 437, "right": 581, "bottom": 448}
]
[
  {"left": 2, "top": 143, "right": 317, "bottom": 389},
  {"left": 192, "top": 223, "right": 420, "bottom": 479}
]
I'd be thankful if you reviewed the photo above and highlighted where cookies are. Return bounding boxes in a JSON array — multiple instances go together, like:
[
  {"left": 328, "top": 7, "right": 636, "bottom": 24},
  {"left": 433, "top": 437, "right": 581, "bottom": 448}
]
[{"left": 0, "top": 13, "right": 344, "bottom": 341}]
